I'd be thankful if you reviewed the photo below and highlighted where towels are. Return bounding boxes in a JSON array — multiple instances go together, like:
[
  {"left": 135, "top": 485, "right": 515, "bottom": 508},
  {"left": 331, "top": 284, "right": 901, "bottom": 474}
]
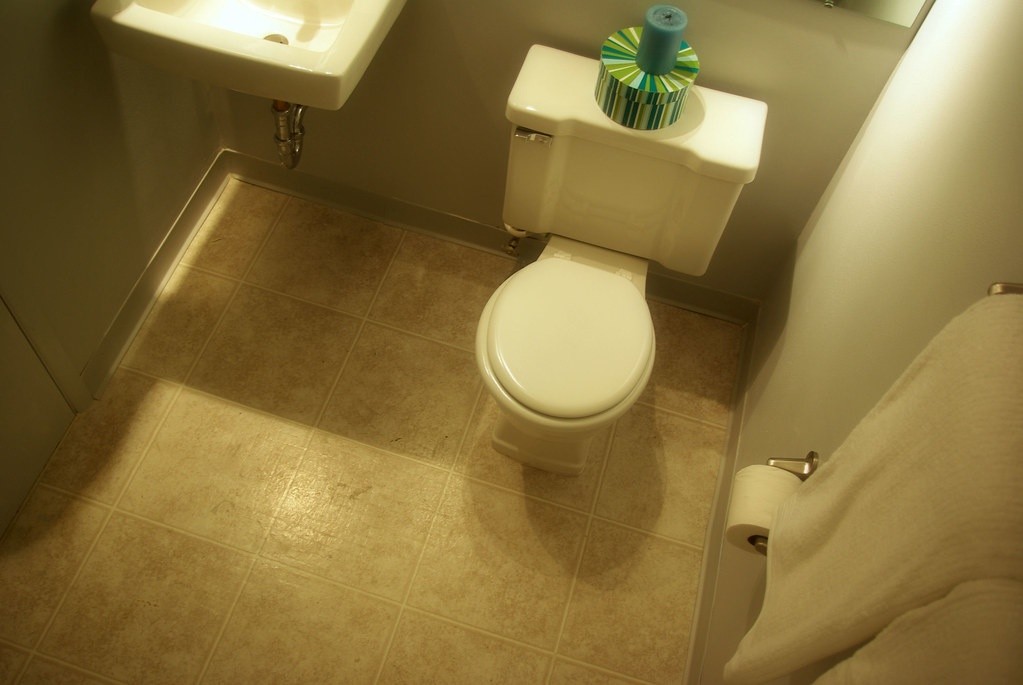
[
  {"left": 809, "top": 577, "right": 1023, "bottom": 685},
  {"left": 720, "top": 291, "right": 1023, "bottom": 685}
]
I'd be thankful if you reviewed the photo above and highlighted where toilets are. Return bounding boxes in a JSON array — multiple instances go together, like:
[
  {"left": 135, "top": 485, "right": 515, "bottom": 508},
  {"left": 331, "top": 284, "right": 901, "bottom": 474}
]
[{"left": 474, "top": 43, "right": 770, "bottom": 478}]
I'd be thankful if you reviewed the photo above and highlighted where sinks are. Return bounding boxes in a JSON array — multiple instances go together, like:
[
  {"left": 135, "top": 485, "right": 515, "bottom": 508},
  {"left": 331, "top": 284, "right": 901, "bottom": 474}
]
[{"left": 90, "top": 0, "right": 408, "bottom": 112}]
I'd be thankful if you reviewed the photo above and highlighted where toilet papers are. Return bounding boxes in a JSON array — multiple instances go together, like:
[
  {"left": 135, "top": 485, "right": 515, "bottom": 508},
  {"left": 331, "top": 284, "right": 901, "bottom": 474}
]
[{"left": 723, "top": 464, "right": 804, "bottom": 558}]
